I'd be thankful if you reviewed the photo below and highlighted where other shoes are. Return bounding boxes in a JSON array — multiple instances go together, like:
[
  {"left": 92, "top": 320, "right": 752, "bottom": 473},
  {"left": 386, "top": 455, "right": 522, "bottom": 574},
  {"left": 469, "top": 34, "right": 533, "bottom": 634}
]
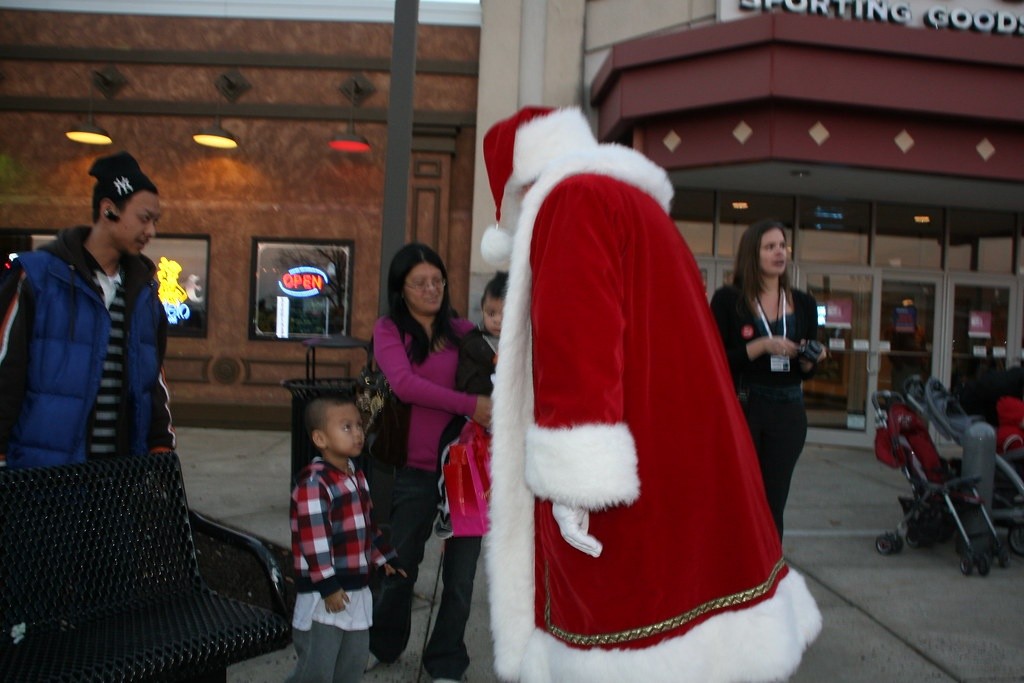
[{"left": 365, "top": 651, "right": 381, "bottom": 673}]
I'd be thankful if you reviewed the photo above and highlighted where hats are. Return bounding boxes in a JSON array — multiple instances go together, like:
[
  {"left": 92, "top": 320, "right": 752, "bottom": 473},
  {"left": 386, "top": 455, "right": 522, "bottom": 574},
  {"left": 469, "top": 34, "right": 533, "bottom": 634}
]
[
  {"left": 481, "top": 104, "right": 600, "bottom": 271},
  {"left": 87, "top": 153, "right": 159, "bottom": 208}
]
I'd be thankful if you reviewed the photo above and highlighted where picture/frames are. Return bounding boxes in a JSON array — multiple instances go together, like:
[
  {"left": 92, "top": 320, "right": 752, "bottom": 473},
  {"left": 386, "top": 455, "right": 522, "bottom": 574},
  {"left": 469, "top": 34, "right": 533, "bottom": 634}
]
[
  {"left": 138, "top": 232, "right": 211, "bottom": 340},
  {"left": 0, "top": 226, "right": 63, "bottom": 265},
  {"left": 249, "top": 236, "right": 354, "bottom": 339}
]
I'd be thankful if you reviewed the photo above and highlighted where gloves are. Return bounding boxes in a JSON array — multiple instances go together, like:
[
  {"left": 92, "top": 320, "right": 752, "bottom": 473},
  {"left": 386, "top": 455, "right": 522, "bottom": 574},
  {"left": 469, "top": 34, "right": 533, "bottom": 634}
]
[{"left": 550, "top": 498, "right": 601, "bottom": 557}]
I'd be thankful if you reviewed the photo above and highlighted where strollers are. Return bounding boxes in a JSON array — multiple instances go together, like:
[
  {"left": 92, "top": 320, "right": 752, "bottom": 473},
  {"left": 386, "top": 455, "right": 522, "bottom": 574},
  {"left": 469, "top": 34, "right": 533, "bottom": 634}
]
[
  {"left": 871, "top": 388, "right": 1010, "bottom": 577},
  {"left": 901, "top": 375, "right": 1024, "bottom": 558}
]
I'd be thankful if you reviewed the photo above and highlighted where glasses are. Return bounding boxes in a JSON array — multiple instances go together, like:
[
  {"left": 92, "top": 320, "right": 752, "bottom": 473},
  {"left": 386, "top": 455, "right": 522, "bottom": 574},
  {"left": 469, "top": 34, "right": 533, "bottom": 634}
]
[{"left": 405, "top": 277, "right": 446, "bottom": 293}]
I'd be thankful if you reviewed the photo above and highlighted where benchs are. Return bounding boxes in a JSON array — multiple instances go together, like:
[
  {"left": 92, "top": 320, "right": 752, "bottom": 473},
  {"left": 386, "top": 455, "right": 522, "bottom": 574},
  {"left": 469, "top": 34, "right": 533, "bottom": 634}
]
[{"left": 0, "top": 445, "right": 297, "bottom": 683}]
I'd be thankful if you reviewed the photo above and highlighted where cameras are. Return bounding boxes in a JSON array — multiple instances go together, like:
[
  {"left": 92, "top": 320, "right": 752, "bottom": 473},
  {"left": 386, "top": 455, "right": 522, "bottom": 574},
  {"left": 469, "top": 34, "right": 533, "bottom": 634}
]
[{"left": 799, "top": 340, "right": 822, "bottom": 364}]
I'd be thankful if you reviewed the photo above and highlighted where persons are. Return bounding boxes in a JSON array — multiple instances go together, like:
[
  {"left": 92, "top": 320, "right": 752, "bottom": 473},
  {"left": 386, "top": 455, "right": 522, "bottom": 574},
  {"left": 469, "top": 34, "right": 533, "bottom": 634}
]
[
  {"left": 1, "top": 152, "right": 175, "bottom": 476},
  {"left": 704, "top": 218, "right": 818, "bottom": 551},
  {"left": 363, "top": 242, "right": 482, "bottom": 683},
  {"left": 472, "top": 103, "right": 824, "bottom": 682},
  {"left": 288, "top": 395, "right": 407, "bottom": 683},
  {"left": 434, "top": 270, "right": 507, "bottom": 540}
]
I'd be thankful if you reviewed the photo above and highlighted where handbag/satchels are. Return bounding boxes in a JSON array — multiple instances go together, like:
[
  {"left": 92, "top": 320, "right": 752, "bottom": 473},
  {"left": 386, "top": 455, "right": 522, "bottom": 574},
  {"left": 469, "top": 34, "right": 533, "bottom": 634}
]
[
  {"left": 351, "top": 315, "right": 408, "bottom": 464},
  {"left": 444, "top": 418, "right": 493, "bottom": 538}
]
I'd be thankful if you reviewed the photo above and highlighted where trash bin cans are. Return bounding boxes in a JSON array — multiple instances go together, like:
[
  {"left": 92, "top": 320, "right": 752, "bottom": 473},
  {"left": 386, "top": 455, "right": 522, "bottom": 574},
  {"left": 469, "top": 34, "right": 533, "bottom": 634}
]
[{"left": 283, "top": 336, "right": 372, "bottom": 518}]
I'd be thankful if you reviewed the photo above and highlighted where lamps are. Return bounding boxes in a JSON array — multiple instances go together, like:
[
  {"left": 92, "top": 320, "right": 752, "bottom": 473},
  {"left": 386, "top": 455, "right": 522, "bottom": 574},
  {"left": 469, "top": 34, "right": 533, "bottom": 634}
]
[
  {"left": 65, "top": 66, "right": 126, "bottom": 147},
  {"left": 192, "top": 69, "right": 249, "bottom": 151},
  {"left": 325, "top": 72, "right": 375, "bottom": 155}
]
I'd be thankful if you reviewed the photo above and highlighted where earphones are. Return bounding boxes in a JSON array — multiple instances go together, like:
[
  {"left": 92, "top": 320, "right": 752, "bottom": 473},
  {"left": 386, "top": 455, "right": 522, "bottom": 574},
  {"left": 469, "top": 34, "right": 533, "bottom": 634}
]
[{"left": 103, "top": 208, "right": 120, "bottom": 223}]
